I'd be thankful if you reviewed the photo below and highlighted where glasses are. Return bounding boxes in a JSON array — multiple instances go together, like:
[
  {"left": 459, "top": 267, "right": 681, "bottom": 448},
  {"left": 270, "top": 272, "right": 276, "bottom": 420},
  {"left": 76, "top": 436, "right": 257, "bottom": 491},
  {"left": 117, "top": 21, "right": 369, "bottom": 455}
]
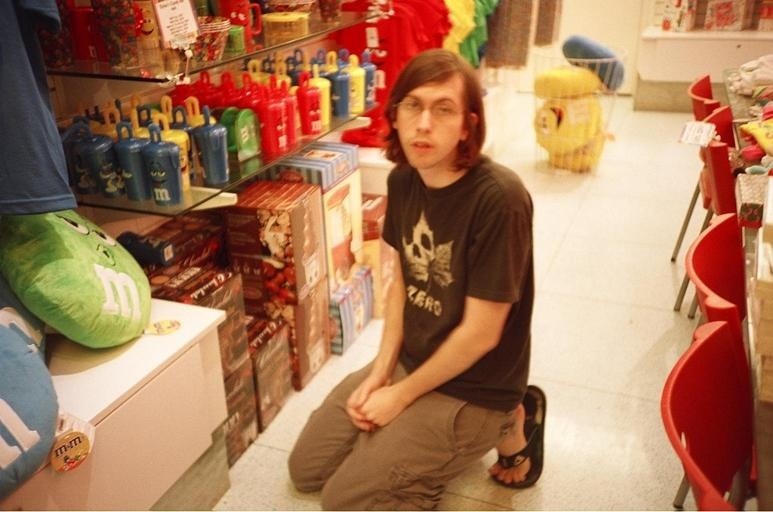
[{"left": 394, "top": 100, "right": 462, "bottom": 118}]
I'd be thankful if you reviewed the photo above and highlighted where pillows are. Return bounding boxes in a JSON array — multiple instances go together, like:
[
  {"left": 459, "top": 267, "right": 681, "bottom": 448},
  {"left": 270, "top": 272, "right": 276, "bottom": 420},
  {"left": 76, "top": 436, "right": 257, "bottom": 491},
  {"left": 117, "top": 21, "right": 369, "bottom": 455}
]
[{"left": 533, "top": 31, "right": 624, "bottom": 173}]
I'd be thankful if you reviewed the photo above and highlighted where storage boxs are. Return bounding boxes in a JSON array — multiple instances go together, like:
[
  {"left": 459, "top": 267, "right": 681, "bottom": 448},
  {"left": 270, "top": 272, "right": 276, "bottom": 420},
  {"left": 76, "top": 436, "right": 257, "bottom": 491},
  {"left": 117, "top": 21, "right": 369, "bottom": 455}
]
[
  {"left": 261, "top": 142, "right": 364, "bottom": 296},
  {"left": 330, "top": 269, "right": 374, "bottom": 356},
  {"left": 141, "top": 268, "right": 250, "bottom": 381},
  {"left": 135, "top": 214, "right": 225, "bottom": 271},
  {"left": 246, "top": 315, "right": 291, "bottom": 433},
  {"left": 224, "top": 359, "right": 261, "bottom": 469},
  {"left": 245, "top": 275, "right": 331, "bottom": 392}
]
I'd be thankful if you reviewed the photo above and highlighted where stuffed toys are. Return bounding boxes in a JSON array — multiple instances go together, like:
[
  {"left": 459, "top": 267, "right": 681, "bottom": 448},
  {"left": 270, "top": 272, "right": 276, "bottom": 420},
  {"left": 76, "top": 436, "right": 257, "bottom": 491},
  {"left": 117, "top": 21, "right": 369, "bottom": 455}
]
[
  {"left": 0, "top": 282, "right": 46, "bottom": 363},
  {"left": 535, "top": 66, "right": 601, "bottom": 98},
  {"left": 548, "top": 136, "right": 605, "bottom": 172},
  {"left": 534, "top": 99, "right": 604, "bottom": 155},
  {"left": 563, "top": 35, "right": 624, "bottom": 91}
]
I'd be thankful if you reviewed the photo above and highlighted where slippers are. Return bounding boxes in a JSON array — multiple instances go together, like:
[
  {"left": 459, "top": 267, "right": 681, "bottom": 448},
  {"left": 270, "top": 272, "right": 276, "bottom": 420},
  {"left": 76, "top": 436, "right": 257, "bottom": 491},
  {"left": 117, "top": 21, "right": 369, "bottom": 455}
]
[{"left": 492, "top": 385, "right": 546, "bottom": 488}]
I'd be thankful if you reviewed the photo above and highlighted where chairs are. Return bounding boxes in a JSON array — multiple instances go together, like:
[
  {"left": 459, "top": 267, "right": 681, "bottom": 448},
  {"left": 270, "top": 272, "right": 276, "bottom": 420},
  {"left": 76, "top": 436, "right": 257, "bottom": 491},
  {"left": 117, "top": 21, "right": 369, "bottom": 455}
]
[
  {"left": 673, "top": 74, "right": 723, "bottom": 261},
  {"left": 684, "top": 215, "right": 747, "bottom": 325},
  {"left": 661, "top": 321, "right": 752, "bottom": 512},
  {"left": 675, "top": 109, "right": 741, "bottom": 319}
]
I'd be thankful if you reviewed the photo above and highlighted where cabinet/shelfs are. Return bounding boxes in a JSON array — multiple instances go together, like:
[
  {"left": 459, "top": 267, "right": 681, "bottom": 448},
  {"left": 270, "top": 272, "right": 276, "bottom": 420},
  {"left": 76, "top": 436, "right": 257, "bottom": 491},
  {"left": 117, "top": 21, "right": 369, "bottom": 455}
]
[
  {"left": 46, "top": 12, "right": 379, "bottom": 214},
  {"left": 632, "top": 24, "right": 773, "bottom": 111},
  {"left": 0, "top": 298, "right": 227, "bottom": 512}
]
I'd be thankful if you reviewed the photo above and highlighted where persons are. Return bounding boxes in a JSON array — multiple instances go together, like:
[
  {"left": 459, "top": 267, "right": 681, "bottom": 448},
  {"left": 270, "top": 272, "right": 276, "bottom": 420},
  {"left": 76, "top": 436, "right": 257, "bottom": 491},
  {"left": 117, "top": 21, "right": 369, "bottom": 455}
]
[{"left": 290, "top": 50, "right": 547, "bottom": 510}]
